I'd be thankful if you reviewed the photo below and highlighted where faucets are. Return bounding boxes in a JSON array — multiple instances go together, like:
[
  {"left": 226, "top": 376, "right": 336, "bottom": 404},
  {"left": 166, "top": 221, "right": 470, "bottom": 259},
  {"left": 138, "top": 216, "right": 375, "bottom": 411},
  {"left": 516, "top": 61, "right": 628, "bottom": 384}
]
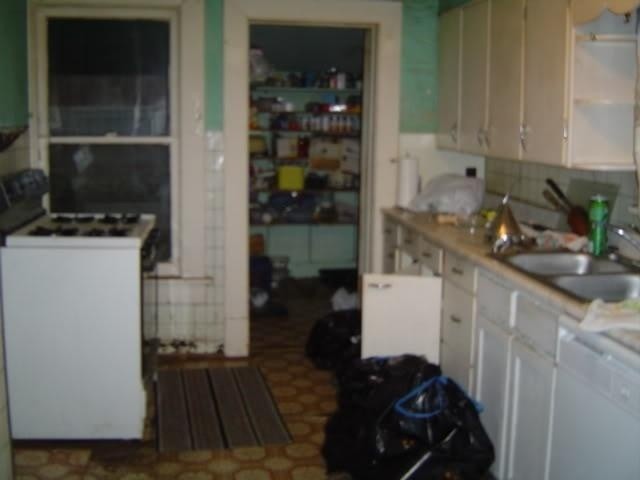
[{"left": 591, "top": 219, "right": 640, "bottom": 252}]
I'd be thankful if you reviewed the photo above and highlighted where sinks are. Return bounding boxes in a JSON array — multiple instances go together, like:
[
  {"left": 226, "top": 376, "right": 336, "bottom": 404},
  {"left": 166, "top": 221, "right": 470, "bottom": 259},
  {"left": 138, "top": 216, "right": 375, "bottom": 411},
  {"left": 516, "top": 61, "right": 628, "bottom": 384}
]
[
  {"left": 484, "top": 249, "right": 627, "bottom": 274},
  {"left": 546, "top": 274, "right": 640, "bottom": 303}
]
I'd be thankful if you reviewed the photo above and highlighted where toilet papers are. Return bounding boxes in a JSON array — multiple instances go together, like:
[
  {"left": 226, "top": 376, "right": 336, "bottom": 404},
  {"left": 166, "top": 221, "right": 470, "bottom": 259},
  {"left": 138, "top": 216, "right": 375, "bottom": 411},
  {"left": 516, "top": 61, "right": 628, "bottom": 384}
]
[{"left": 396, "top": 158, "right": 419, "bottom": 209}]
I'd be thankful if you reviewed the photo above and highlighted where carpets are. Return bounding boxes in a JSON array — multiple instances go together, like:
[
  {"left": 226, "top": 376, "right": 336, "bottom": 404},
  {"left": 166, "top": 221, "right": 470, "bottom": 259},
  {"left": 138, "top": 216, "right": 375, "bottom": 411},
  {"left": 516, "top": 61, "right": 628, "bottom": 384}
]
[{"left": 91, "top": 364, "right": 294, "bottom": 460}]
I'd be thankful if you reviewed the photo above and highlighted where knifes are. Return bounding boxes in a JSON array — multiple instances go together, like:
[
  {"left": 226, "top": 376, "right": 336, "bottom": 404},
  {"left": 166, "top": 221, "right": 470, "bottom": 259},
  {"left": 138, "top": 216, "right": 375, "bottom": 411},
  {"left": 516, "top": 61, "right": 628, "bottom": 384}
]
[{"left": 543, "top": 177, "right": 573, "bottom": 214}]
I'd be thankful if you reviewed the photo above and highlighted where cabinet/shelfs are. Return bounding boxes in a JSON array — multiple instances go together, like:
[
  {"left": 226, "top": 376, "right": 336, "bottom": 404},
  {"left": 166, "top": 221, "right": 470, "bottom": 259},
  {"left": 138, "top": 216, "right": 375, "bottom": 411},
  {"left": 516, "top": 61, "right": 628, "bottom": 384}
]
[
  {"left": 250, "top": 224, "right": 358, "bottom": 276},
  {"left": 403, "top": 210, "right": 455, "bottom": 277},
  {"left": 249, "top": 84, "right": 362, "bottom": 191},
  {"left": 550, "top": 298, "right": 639, "bottom": 479},
  {"left": 434, "top": 0, "right": 491, "bottom": 154},
  {"left": 378, "top": 211, "right": 401, "bottom": 275},
  {"left": 491, "top": 0, "right": 640, "bottom": 171},
  {"left": 475, "top": 258, "right": 559, "bottom": 479},
  {"left": 437, "top": 213, "right": 480, "bottom": 400}
]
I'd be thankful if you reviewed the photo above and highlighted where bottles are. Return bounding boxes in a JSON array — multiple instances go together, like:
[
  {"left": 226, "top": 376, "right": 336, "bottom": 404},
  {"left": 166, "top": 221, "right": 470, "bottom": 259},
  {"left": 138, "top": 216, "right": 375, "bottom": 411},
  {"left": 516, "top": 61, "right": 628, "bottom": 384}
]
[
  {"left": 329, "top": 68, "right": 337, "bottom": 88},
  {"left": 588, "top": 195, "right": 609, "bottom": 256}
]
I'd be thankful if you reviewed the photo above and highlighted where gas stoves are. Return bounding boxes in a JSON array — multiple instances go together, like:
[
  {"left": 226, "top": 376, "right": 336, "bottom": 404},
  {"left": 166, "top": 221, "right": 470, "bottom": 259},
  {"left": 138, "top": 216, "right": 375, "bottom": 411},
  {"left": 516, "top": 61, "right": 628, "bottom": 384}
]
[{"left": 3, "top": 209, "right": 158, "bottom": 248}]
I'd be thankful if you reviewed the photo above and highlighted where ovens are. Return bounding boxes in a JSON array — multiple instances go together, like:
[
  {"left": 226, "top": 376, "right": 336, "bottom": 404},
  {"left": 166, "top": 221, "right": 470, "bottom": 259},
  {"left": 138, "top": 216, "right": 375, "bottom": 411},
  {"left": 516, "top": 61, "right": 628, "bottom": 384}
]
[{"left": 140, "top": 225, "right": 160, "bottom": 400}]
